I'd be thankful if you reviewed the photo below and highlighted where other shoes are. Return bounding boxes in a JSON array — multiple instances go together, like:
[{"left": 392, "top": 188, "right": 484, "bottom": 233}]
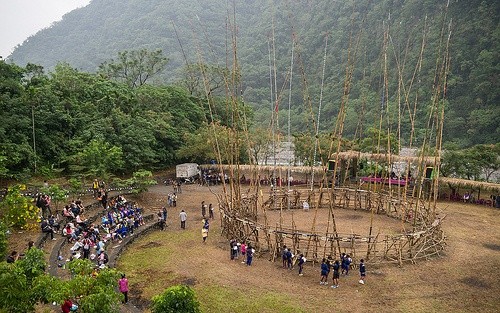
[
  {"left": 331, "top": 285, "right": 336, "bottom": 288},
  {"left": 336, "top": 285, "right": 339, "bottom": 287},
  {"left": 298, "top": 274, "right": 303, "bottom": 276},
  {"left": 358, "top": 279, "right": 364, "bottom": 285},
  {"left": 319, "top": 281, "right": 328, "bottom": 285}
]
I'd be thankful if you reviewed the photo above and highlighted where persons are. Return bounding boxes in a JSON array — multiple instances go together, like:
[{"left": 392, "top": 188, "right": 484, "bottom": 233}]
[
  {"left": 463, "top": 192, "right": 469, "bottom": 202},
  {"left": 156, "top": 207, "right": 167, "bottom": 231},
  {"left": 164, "top": 165, "right": 229, "bottom": 193},
  {"left": 201, "top": 201, "right": 213, "bottom": 243},
  {"left": 367, "top": 170, "right": 412, "bottom": 180},
  {"left": 256, "top": 175, "right": 294, "bottom": 187},
  {"left": 118, "top": 273, "right": 129, "bottom": 304},
  {"left": 359, "top": 259, "right": 365, "bottom": 284},
  {"left": 62, "top": 298, "right": 74, "bottom": 313},
  {"left": 28, "top": 238, "right": 34, "bottom": 248},
  {"left": 340, "top": 253, "right": 352, "bottom": 275},
  {"left": 320, "top": 255, "right": 339, "bottom": 288},
  {"left": 281, "top": 246, "right": 307, "bottom": 277},
  {"left": 179, "top": 210, "right": 187, "bottom": 229},
  {"left": 230, "top": 239, "right": 255, "bottom": 265},
  {"left": 168, "top": 192, "right": 177, "bottom": 207},
  {"left": 7, "top": 251, "right": 19, "bottom": 264},
  {"left": 240, "top": 174, "right": 246, "bottom": 183},
  {"left": 35, "top": 178, "right": 145, "bottom": 278}
]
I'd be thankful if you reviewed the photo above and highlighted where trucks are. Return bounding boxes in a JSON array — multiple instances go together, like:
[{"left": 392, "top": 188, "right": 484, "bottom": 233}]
[{"left": 176, "top": 163, "right": 202, "bottom": 182}]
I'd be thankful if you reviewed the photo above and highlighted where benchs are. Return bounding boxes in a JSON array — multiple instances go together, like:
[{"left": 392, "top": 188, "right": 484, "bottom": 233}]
[{"left": 28, "top": 196, "right": 166, "bottom": 313}]
